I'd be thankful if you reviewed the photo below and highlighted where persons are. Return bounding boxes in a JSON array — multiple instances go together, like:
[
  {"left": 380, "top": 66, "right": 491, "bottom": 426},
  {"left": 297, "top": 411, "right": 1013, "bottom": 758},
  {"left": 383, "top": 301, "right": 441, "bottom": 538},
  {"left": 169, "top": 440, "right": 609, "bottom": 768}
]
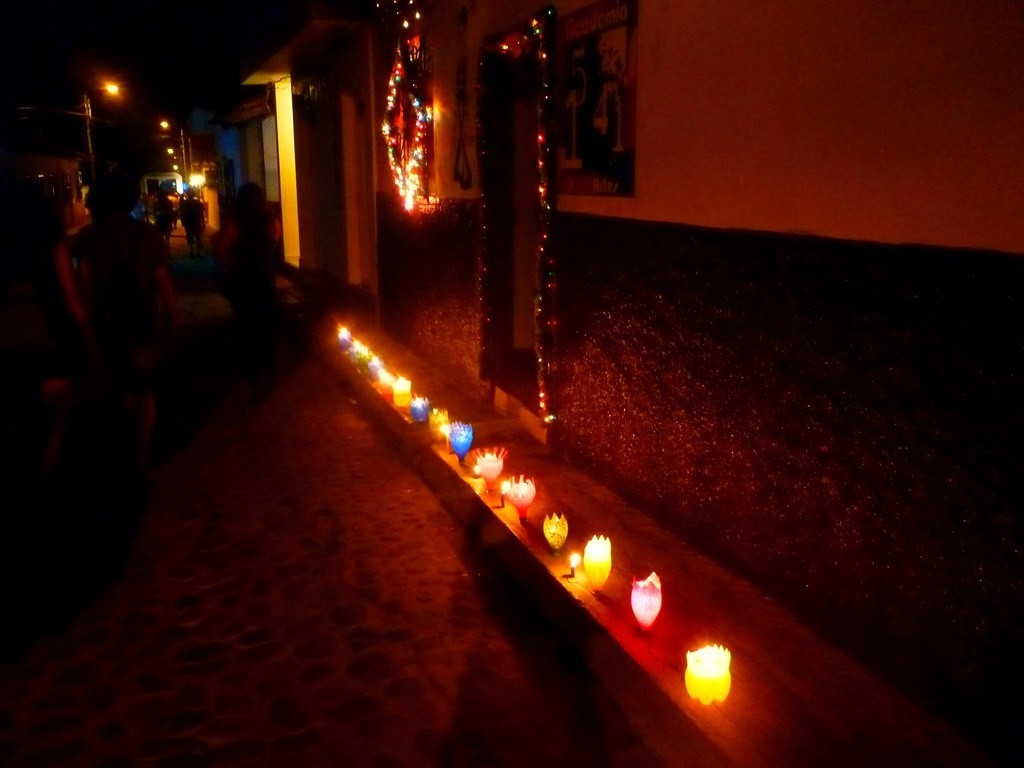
[
  {"left": 229, "top": 182, "right": 274, "bottom": 311},
  {"left": 39, "top": 174, "right": 179, "bottom": 488},
  {"left": 134, "top": 182, "right": 206, "bottom": 260}
]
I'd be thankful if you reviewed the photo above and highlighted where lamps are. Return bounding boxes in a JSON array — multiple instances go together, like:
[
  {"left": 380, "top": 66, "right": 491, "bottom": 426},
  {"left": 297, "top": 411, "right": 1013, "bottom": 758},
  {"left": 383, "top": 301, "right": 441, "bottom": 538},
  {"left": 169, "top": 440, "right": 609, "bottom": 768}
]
[
  {"left": 336, "top": 326, "right": 536, "bottom": 521},
  {"left": 569, "top": 553, "right": 582, "bottom": 576},
  {"left": 632, "top": 572, "right": 662, "bottom": 631},
  {"left": 543, "top": 512, "right": 569, "bottom": 556},
  {"left": 583, "top": 535, "right": 613, "bottom": 591},
  {"left": 685, "top": 644, "right": 731, "bottom": 705}
]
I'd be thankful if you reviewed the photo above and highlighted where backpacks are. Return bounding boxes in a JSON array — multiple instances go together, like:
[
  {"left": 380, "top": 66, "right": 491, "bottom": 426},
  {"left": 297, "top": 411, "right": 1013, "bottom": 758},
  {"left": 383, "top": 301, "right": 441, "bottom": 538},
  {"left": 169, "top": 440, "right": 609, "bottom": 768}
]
[
  {"left": 96, "top": 222, "right": 154, "bottom": 332},
  {"left": 228, "top": 216, "right": 273, "bottom": 303},
  {"left": 0, "top": 246, "right": 58, "bottom": 356}
]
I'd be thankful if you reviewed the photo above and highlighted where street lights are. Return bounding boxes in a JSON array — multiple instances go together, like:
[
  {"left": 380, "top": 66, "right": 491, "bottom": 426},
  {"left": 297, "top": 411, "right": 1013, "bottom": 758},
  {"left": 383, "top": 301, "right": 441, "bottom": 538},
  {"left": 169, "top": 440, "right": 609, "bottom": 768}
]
[
  {"left": 160, "top": 119, "right": 189, "bottom": 186},
  {"left": 79, "top": 81, "right": 118, "bottom": 180}
]
